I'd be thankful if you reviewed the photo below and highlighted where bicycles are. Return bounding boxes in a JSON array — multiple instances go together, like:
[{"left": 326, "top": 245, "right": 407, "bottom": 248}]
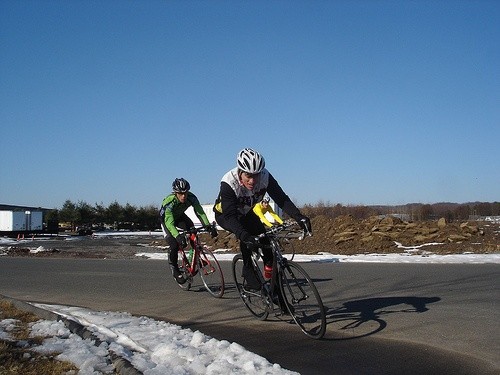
[
  {"left": 229, "top": 217, "right": 328, "bottom": 341},
  {"left": 166, "top": 219, "right": 226, "bottom": 300}
]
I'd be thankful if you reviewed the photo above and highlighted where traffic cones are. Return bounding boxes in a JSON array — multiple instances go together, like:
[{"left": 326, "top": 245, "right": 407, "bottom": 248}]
[
  {"left": 21, "top": 234, "right": 25, "bottom": 240},
  {"left": 31, "top": 236, "right": 35, "bottom": 241},
  {"left": 16, "top": 234, "right": 20, "bottom": 242}
]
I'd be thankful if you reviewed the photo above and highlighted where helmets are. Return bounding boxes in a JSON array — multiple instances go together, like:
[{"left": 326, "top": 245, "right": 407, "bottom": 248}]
[
  {"left": 237, "top": 148, "right": 265, "bottom": 175},
  {"left": 172, "top": 178, "right": 190, "bottom": 193},
  {"left": 262, "top": 197, "right": 270, "bottom": 203}
]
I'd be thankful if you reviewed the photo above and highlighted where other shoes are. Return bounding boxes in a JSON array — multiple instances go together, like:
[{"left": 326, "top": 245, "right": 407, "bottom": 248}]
[
  {"left": 242, "top": 265, "right": 262, "bottom": 290},
  {"left": 273, "top": 297, "right": 295, "bottom": 315}
]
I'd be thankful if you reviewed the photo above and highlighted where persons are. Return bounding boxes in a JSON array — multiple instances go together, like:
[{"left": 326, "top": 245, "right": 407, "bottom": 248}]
[
  {"left": 159, "top": 178, "right": 217, "bottom": 284},
  {"left": 213, "top": 148, "right": 312, "bottom": 315},
  {"left": 252, "top": 197, "right": 285, "bottom": 230}
]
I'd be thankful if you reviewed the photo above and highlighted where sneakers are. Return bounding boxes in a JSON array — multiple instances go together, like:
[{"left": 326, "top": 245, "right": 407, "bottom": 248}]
[
  {"left": 175, "top": 276, "right": 186, "bottom": 285},
  {"left": 198, "top": 259, "right": 207, "bottom": 268}
]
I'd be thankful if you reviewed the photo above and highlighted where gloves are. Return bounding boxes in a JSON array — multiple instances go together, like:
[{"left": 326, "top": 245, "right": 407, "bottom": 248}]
[
  {"left": 295, "top": 213, "right": 312, "bottom": 232},
  {"left": 242, "top": 233, "right": 259, "bottom": 251},
  {"left": 175, "top": 235, "right": 187, "bottom": 248},
  {"left": 209, "top": 228, "right": 218, "bottom": 238}
]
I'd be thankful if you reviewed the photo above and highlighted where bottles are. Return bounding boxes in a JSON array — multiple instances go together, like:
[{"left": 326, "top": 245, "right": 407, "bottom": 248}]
[
  {"left": 263, "top": 262, "right": 273, "bottom": 282},
  {"left": 188, "top": 247, "right": 194, "bottom": 263}
]
